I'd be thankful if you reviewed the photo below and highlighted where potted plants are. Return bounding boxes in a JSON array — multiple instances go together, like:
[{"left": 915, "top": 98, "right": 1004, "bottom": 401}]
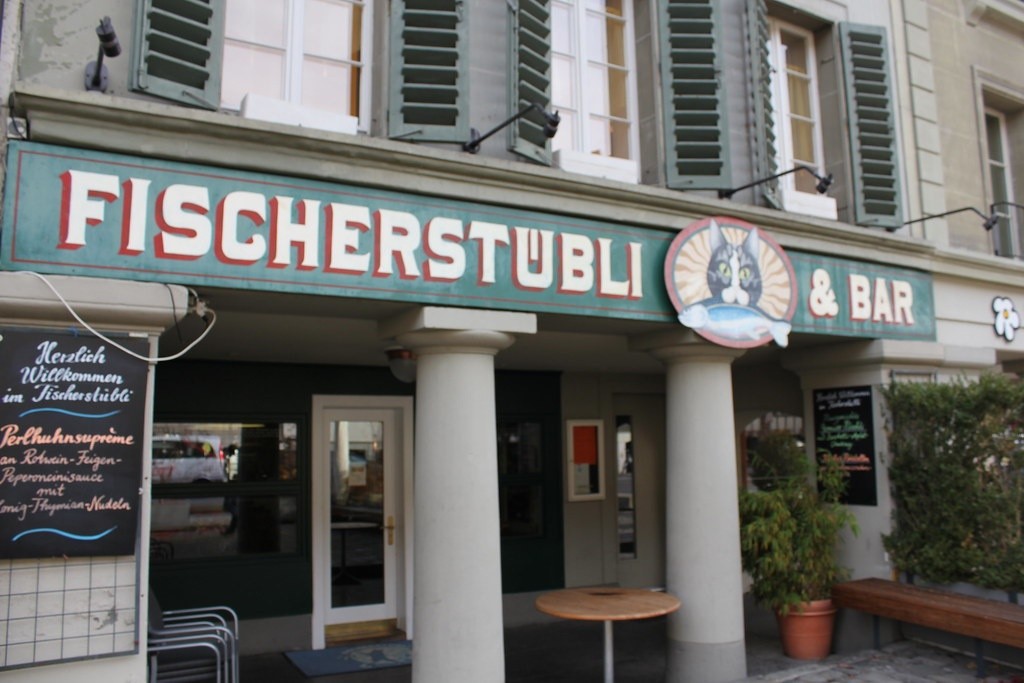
[{"left": 740, "top": 461, "right": 857, "bottom": 662}]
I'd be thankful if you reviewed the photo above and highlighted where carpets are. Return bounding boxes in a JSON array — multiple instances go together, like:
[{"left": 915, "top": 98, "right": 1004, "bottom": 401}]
[{"left": 282, "top": 639, "right": 414, "bottom": 678}]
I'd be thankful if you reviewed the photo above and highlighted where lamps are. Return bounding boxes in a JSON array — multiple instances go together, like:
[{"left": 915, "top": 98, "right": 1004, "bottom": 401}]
[
  {"left": 885, "top": 203, "right": 998, "bottom": 234},
  {"left": 720, "top": 165, "right": 834, "bottom": 203},
  {"left": 462, "top": 102, "right": 563, "bottom": 157},
  {"left": 87, "top": 17, "right": 122, "bottom": 92}
]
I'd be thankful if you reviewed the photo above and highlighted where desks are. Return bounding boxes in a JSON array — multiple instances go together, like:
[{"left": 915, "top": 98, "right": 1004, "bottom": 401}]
[{"left": 537, "top": 589, "right": 682, "bottom": 683}]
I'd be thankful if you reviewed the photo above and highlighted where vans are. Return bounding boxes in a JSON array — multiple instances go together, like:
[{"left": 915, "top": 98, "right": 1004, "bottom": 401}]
[{"left": 152, "top": 435, "right": 226, "bottom": 487}]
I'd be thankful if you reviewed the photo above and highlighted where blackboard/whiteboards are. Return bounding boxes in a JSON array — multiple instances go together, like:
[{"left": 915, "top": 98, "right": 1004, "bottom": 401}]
[
  {"left": 813, "top": 385, "right": 877, "bottom": 506},
  {"left": 0, "top": 324, "right": 150, "bottom": 559}
]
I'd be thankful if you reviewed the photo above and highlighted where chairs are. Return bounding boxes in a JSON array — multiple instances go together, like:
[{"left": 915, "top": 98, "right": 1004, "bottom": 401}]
[{"left": 148, "top": 589, "right": 240, "bottom": 682}]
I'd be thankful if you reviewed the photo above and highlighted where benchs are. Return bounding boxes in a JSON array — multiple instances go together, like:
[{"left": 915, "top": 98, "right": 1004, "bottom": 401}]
[{"left": 834, "top": 576, "right": 1024, "bottom": 683}]
